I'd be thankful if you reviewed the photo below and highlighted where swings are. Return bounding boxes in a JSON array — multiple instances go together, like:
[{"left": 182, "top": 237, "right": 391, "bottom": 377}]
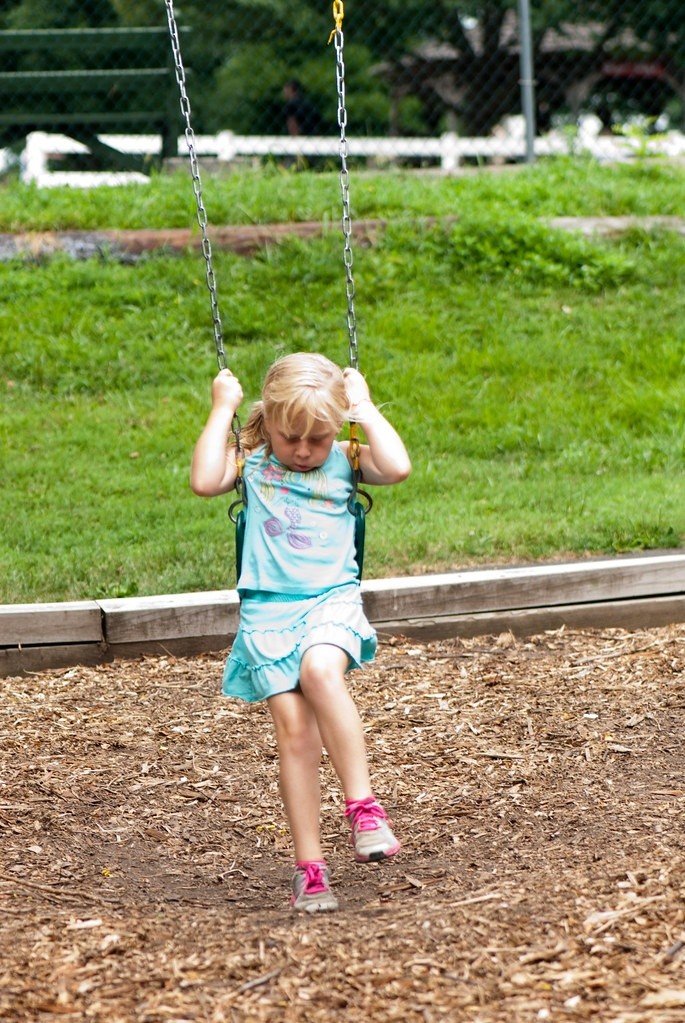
[{"left": 166, "top": 1, "right": 367, "bottom": 589}]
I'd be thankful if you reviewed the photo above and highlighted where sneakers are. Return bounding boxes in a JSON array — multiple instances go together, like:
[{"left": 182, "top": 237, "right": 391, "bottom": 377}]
[
  {"left": 344, "top": 797, "right": 400, "bottom": 862},
  {"left": 290, "top": 860, "right": 338, "bottom": 913}
]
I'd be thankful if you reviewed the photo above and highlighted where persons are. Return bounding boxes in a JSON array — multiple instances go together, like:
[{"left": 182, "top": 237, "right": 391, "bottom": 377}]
[{"left": 190, "top": 351, "right": 412, "bottom": 914}]
